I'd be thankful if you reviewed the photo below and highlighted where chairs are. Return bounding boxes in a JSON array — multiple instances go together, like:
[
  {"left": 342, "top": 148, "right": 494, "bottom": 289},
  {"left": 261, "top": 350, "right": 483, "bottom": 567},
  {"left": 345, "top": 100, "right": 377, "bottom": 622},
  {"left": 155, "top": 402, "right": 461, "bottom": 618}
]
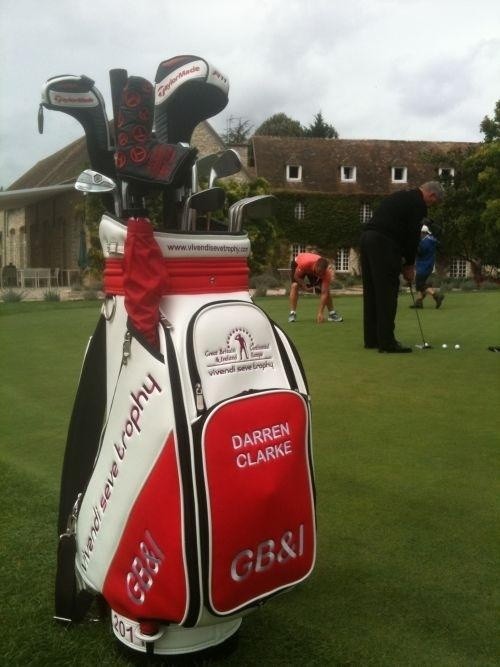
[{"left": 16, "top": 267, "right": 60, "bottom": 290}]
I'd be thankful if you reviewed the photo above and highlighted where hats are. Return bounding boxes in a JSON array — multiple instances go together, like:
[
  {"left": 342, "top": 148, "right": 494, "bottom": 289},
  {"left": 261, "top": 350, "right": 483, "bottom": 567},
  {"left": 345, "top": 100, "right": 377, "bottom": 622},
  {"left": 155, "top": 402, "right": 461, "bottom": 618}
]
[{"left": 421, "top": 225, "right": 432, "bottom": 235}]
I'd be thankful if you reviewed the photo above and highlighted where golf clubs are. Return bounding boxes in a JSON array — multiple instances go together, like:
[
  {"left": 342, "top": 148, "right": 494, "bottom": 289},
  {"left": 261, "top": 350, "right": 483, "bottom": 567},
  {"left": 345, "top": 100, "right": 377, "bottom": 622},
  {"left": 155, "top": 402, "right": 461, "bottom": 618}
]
[
  {"left": 408, "top": 282, "right": 432, "bottom": 349},
  {"left": 38, "top": 54, "right": 280, "bottom": 234}
]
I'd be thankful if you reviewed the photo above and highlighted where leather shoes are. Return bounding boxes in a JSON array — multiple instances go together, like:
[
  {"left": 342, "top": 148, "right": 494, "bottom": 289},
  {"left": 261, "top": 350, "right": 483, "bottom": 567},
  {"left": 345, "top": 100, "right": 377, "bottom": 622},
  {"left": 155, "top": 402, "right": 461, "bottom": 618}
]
[{"left": 365, "top": 342, "right": 412, "bottom": 353}]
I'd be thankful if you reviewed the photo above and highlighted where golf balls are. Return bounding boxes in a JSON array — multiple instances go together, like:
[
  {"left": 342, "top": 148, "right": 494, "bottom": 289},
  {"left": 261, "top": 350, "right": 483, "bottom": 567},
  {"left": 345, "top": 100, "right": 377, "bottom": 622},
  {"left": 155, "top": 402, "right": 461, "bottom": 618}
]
[
  {"left": 455, "top": 345, "right": 460, "bottom": 350},
  {"left": 442, "top": 344, "right": 447, "bottom": 349},
  {"left": 425, "top": 343, "right": 428, "bottom": 347}
]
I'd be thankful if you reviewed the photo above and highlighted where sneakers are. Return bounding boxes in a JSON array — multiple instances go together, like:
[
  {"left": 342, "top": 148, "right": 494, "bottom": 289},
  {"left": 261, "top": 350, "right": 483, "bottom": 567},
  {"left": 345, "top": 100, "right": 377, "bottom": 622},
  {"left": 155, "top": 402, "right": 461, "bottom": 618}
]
[
  {"left": 288, "top": 312, "right": 296, "bottom": 322},
  {"left": 410, "top": 304, "right": 423, "bottom": 308},
  {"left": 436, "top": 295, "right": 444, "bottom": 308},
  {"left": 328, "top": 313, "right": 343, "bottom": 322}
]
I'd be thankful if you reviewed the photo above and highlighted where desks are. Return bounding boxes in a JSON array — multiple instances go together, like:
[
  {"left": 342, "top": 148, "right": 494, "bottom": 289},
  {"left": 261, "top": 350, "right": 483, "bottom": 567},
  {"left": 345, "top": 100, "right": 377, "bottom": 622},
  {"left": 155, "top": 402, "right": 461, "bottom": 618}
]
[{"left": 61, "top": 269, "right": 81, "bottom": 287}]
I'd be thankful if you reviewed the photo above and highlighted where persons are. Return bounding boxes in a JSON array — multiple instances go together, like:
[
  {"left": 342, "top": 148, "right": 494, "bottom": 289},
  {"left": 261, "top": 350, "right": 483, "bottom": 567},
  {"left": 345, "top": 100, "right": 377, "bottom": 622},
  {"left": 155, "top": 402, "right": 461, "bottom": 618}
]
[
  {"left": 358, "top": 178, "right": 446, "bottom": 355},
  {"left": 411, "top": 223, "right": 444, "bottom": 309},
  {"left": 288, "top": 251, "right": 344, "bottom": 323}
]
